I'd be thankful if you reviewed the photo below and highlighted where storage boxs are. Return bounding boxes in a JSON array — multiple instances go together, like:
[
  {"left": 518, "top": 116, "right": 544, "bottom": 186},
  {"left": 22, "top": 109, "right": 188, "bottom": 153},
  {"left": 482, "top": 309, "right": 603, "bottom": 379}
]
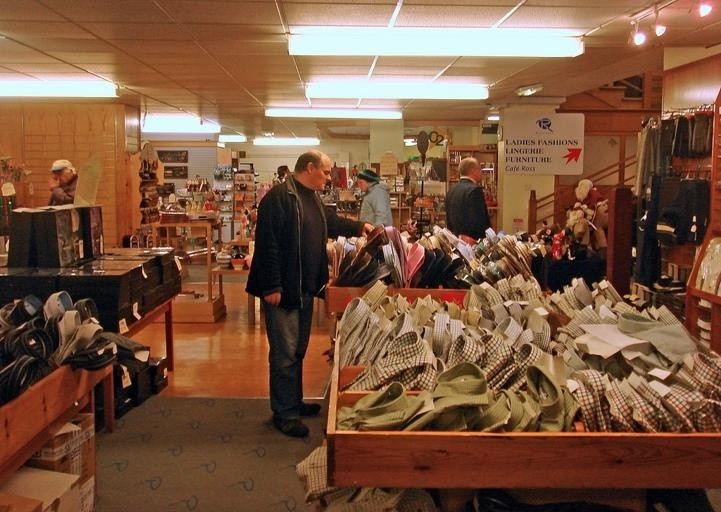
[
  {"left": 1, "top": 197, "right": 184, "bottom": 334},
  {"left": 0, "top": 412, "right": 98, "bottom": 512}
]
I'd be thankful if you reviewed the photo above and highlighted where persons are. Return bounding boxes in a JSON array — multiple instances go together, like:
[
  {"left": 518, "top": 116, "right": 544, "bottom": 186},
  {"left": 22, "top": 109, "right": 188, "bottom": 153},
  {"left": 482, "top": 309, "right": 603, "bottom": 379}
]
[
  {"left": 355, "top": 169, "right": 394, "bottom": 229},
  {"left": 245, "top": 149, "right": 370, "bottom": 438},
  {"left": 445, "top": 155, "right": 493, "bottom": 243},
  {"left": 53, "top": 159, "right": 79, "bottom": 207},
  {"left": 276, "top": 164, "right": 290, "bottom": 183}
]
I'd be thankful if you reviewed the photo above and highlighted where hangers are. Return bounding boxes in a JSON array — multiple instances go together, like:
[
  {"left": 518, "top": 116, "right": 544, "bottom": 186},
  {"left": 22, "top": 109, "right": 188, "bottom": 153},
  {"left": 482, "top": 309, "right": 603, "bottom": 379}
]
[{"left": 641, "top": 103, "right": 712, "bottom": 190}]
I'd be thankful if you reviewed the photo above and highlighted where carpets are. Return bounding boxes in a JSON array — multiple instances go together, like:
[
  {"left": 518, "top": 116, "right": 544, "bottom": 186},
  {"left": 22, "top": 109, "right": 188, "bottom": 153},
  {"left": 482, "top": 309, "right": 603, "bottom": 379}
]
[{"left": 90, "top": 397, "right": 326, "bottom": 512}]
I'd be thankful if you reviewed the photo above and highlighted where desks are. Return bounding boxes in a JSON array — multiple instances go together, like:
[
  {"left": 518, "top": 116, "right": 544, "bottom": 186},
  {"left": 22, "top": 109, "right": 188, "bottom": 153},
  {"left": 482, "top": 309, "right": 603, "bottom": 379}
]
[
  {"left": 211, "top": 265, "right": 261, "bottom": 329},
  {"left": 2, "top": 351, "right": 115, "bottom": 488},
  {"left": 119, "top": 293, "right": 182, "bottom": 371}
]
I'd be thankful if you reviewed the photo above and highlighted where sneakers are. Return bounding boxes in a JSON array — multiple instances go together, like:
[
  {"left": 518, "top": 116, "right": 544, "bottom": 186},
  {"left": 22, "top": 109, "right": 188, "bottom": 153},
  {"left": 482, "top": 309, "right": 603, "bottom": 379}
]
[
  {"left": 296, "top": 400, "right": 322, "bottom": 416},
  {"left": 272, "top": 413, "right": 310, "bottom": 438}
]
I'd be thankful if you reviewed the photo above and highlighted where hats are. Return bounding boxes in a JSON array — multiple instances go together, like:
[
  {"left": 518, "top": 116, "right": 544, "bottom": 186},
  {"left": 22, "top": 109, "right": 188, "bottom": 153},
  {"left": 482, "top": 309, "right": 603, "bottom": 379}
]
[
  {"left": 357, "top": 170, "right": 379, "bottom": 183},
  {"left": 50, "top": 159, "right": 73, "bottom": 173}
]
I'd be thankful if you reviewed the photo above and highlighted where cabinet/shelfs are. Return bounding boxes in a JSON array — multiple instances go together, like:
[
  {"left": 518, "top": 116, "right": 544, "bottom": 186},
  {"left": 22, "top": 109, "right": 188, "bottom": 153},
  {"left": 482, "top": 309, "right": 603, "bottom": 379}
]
[
  {"left": 684, "top": 86, "right": 720, "bottom": 358},
  {"left": 210, "top": 143, "right": 498, "bottom": 253},
  {"left": 147, "top": 215, "right": 226, "bottom": 323}
]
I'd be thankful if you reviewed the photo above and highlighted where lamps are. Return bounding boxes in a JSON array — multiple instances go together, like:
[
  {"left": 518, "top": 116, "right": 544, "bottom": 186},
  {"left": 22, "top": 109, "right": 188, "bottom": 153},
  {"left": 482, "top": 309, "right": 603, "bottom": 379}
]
[{"left": 628, "top": 0, "right": 715, "bottom": 48}]
